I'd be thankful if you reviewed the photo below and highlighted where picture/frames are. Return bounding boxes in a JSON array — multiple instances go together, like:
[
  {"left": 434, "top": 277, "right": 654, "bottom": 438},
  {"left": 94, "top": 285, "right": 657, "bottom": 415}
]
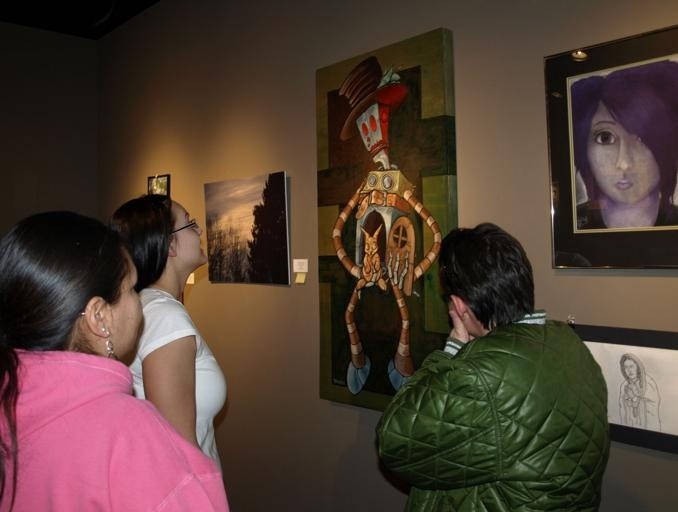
[
  {"left": 148, "top": 173, "right": 170, "bottom": 197},
  {"left": 569, "top": 324, "right": 678, "bottom": 453},
  {"left": 543, "top": 24, "right": 678, "bottom": 270}
]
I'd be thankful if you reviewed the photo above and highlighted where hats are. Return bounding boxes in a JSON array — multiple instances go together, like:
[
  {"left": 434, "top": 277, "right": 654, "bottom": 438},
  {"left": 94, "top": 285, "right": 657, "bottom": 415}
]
[{"left": 337, "top": 54, "right": 412, "bottom": 143}]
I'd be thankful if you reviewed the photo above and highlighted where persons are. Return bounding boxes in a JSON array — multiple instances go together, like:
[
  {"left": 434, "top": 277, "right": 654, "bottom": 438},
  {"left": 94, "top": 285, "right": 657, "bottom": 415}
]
[
  {"left": 570, "top": 59, "right": 678, "bottom": 231},
  {"left": 104, "top": 194, "right": 228, "bottom": 499},
  {"left": 375, "top": 221, "right": 609, "bottom": 512},
  {"left": 1, "top": 207, "right": 229, "bottom": 512}
]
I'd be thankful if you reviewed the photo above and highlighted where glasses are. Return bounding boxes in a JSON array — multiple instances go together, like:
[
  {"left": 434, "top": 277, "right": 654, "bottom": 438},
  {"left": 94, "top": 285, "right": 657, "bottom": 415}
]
[{"left": 166, "top": 217, "right": 198, "bottom": 239}]
